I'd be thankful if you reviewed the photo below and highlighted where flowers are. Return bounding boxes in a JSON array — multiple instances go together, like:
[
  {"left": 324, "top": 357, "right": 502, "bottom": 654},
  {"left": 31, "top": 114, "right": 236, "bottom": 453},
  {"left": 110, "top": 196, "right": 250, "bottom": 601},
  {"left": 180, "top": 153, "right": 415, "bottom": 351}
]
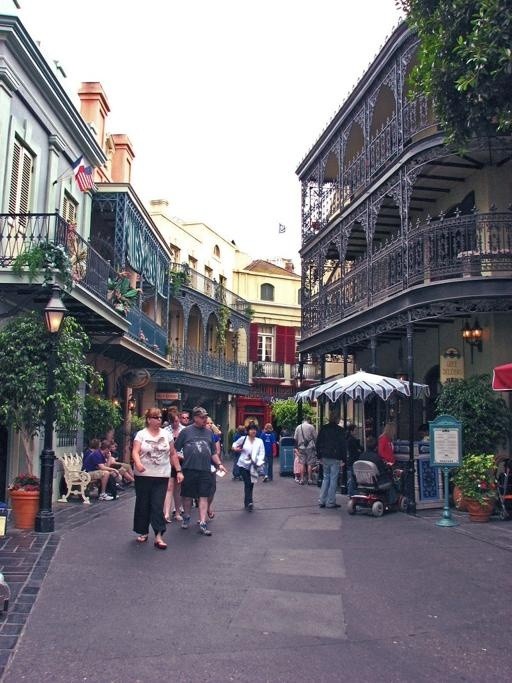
[
  {"left": 7, "top": 472, "right": 39, "bottom": 492},
  {"left": 451, "top": 453, "right": 499, "bottom": 512}
]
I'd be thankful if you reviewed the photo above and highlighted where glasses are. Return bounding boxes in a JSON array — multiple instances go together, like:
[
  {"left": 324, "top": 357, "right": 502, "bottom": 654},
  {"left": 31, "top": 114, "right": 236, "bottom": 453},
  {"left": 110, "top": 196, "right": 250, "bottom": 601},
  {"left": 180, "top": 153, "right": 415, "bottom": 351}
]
[{"left": 151, "top": 416, "right": 163, "bottom": 419}]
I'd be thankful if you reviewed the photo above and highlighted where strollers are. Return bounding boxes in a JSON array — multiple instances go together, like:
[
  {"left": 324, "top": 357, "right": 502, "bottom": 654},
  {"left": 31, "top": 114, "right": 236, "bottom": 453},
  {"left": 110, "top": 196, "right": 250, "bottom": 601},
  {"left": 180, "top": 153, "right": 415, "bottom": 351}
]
[
  {"left": 491, "top": 454, "right": 511, "bottom": 520},
  {"left": 347, "top": 452, "right": 417, "bottom": 517}
]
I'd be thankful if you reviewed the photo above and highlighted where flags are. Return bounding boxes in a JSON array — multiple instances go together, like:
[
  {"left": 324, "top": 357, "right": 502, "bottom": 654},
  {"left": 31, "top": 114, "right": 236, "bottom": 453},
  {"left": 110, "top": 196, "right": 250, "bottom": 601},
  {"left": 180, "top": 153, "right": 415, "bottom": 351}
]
[
  {"left": 71, "top": 154, "right": 90, "bottom": 191},
  {"left": 84, "top": 167, "right": 98, "bottom": 198}
]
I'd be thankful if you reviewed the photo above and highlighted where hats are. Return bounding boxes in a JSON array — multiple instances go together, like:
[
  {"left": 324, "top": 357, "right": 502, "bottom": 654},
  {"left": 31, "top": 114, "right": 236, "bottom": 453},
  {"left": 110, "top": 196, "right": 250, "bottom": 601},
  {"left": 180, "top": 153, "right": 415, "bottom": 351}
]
[{"left": 192, "top": 407, "right": 208, "bottom": 417}]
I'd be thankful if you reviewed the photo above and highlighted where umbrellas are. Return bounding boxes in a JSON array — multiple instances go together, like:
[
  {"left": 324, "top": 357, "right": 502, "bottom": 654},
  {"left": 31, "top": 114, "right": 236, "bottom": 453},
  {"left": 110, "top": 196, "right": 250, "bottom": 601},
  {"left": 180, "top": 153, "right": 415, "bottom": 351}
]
[{"left": 294, "top": 365, "right": 430, "bottom": 453}]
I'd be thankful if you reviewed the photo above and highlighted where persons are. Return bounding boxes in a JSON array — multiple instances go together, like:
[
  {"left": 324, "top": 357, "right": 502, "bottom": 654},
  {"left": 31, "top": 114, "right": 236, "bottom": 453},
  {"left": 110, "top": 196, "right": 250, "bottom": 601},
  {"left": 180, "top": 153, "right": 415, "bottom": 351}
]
[
  {"left": 315, "top": 409, "right": 345, "bottom": 508},
  {"left": 418, "top": 423, "right": 430, "bottom": 442},
  {"left": 294, "top": 416, "right": 318, "bottom": 485},
  {"left": 356, "top": 438, "right": 390, "bottom": 477},
  {"left": 294, "top": 447, "right": 301, "bottom": 483},
  {"left": 344, "top": 423, "right": 363, "bottom": 500},
  {"left": 378, "top": 422, "right": 395, "bottom": 469},
  {"left": 81, "top": 404, "right": 275, "bottom": 549}
]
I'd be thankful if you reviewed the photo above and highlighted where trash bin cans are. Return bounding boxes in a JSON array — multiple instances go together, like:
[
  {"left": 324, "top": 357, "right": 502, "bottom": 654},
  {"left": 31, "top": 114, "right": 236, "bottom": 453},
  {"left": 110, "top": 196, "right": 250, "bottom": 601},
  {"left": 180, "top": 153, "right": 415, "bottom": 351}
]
[{"left": 279, "top": 437, "right": 296, "bottom": 477}]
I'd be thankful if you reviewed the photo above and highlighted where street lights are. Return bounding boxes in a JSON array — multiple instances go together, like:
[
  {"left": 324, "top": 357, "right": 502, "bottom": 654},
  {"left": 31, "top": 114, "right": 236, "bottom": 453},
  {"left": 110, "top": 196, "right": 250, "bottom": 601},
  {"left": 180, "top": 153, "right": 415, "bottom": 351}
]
[{"left": 34, "top": 283, "right": 68, "bottom": 532}]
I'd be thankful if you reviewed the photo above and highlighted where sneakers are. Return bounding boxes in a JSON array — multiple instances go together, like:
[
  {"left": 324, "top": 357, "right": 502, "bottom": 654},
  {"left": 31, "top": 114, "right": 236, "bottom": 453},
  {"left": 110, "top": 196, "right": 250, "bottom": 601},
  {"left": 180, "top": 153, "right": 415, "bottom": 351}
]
[
  {"left": 295, "top": 477, "right": 313, "bottom": 485},
  {"left": 99, "top": 493, "right": 114, "bottom": 501},
  {"left": 163, "top": 498, "right": 214, "bottom": 535},
  {"left": 136, "top": 536, "right": 148, "bottom": 542},
  {"left": 155, "top": 540, "right": 167, "bottom": 548},
  {"left": 262, "top": 475, "right": 268, "bottom": 481},
  {"left": 319, "top": 503, "right": 325, "bottom": 508},
  {"left": 246, "top": 503, "right": 253, "bottom": 512},
  {"left": 114, "top": 484, "right": 124, "bottom": 490},
  {"left": 326, "top": 503, "right": 341, "bottom": 508}
]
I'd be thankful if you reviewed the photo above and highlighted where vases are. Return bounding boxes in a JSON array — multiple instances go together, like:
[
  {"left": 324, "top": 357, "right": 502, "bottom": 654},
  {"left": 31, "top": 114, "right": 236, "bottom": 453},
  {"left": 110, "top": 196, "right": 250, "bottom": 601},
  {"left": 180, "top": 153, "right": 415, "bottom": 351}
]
[
  {"left": 9, "top": 490, "right": 40, "bottom": 529},
  {"left": 462, "top": 498, "right": 496, "bottom": 523}
]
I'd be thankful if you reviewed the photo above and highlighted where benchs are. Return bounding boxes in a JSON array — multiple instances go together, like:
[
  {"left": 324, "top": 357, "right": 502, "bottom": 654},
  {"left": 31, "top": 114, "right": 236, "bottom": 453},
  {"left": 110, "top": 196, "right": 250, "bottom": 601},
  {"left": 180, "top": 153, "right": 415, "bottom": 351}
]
[{"left": 57, "top": 452, "right": 105, "bottom": 504}]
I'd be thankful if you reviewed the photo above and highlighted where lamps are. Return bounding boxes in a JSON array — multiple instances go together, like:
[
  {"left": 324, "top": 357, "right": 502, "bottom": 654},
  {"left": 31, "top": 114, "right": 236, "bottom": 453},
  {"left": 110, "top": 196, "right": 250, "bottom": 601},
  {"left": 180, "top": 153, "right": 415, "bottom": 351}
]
[
  {"left": 128, "top": 391, "right": 136, "bottom": 411},
  {"left": 460, "top": 317, "right": 483, "bottom": 364},
  {"left": 44, "top": 283, "right": 69, "bottom": 333},
  {"left": 112, "top": 391, "right": 120, "bottom": 409}
]
